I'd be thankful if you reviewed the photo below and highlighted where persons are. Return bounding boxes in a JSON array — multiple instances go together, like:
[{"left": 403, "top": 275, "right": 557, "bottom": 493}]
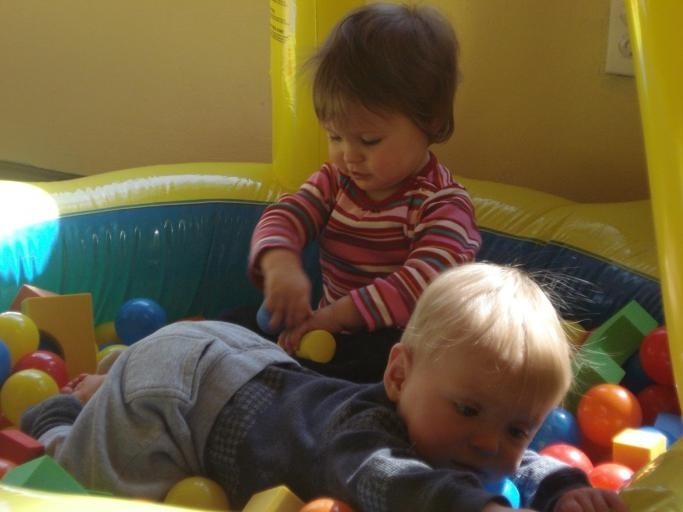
[
  {"left": 20, "top": 263, "right": 631, "bottom": 512},
  {"left": 248, "top": 2, "right": 482, "bottom": 386}
]
